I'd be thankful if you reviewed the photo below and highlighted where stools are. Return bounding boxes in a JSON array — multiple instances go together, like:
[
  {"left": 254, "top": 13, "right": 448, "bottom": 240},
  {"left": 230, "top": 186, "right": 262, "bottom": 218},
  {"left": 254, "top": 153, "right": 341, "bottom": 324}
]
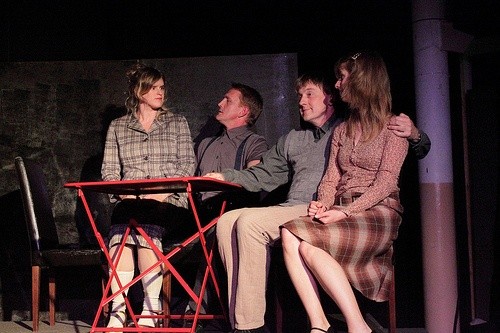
[{"left": 272, "top": 256, "right": 397, "bottom": 333}]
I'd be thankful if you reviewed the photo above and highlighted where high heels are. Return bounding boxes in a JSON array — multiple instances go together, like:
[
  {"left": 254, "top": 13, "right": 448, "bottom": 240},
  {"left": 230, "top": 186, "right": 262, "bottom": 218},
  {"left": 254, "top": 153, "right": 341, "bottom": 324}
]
[
  {"left": 104, "top": 309, "right": 128, "bottom": 333},
  {"left": 134, "top": 303, "right": 166, "bottom": 329}
]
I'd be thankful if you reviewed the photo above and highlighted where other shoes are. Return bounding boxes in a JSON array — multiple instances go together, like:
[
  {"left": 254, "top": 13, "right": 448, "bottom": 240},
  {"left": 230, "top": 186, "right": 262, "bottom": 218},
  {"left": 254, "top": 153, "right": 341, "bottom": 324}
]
[{"left": 186, "top": 311, "right": 209, "bottom": 330}]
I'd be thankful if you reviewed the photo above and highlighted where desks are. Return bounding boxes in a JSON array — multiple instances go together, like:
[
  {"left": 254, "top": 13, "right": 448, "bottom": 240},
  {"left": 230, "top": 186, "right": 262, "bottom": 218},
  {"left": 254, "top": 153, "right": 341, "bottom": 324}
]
[{"left": 63, "top": 175, "right": 246, "bottom": 333}]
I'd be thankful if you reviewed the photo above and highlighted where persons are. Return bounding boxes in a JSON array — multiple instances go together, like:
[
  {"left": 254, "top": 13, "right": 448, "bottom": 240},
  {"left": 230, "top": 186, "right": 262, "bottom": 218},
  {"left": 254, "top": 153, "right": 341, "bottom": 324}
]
[
  {"left": 168, "top": 81, "right": 271, "bottom": 333},
  {"left": 204, "top": 73, "right": 433, "bottom": 333},
  {"left": 279, "top": 50, "right": 412, "bottom": 333},
  {"left": 100, "top": 59, "right": 201, "bottom": 333}
]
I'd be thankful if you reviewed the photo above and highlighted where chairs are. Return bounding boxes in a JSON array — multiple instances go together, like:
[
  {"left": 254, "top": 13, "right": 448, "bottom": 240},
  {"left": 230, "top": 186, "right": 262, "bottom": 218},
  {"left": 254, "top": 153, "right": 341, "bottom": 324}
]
[
  {"left": 14, "top": 155, "right": 117, "bottom": 333},
  {"left": 73, "top": 156, "right": 172, "bottom": 327}
]
